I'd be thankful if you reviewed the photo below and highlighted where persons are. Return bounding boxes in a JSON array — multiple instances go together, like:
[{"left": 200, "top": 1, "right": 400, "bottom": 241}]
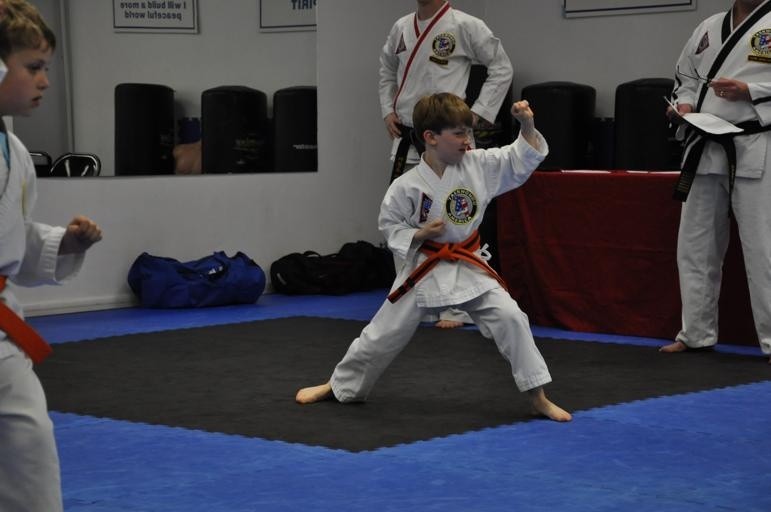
[
  {"left": 379, "top": 1, "right": 514, "bottom": 327},
  {"left": 1, "top": 0, "right": 103, "bottom": 511},
  {"left": 172, "top": 140, "right": 202, "bottom": 173},
  {"left": 659, "top": 1, "right": 770, "bottom": 359},
  {"left": 295, "top": 93, "right": 571, "bottom": 422}
]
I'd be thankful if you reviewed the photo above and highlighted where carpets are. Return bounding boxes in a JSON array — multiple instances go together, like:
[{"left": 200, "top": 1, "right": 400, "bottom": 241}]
[{"left": 35, "top": 315, "right": 771, "bottom": 454}]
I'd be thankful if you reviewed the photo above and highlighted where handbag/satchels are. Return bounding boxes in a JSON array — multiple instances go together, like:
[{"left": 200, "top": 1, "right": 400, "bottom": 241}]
[
  {"left": 128, "top": 251, "right": 267, "bottom": 310},
  {"left": 270, "top": 240, "right": 394, "bottom": 296}
]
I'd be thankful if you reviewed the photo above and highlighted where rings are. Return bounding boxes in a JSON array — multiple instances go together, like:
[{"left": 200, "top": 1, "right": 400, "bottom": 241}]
[{"left": 720, "top": 91, "right": 725, "bottom": 97}]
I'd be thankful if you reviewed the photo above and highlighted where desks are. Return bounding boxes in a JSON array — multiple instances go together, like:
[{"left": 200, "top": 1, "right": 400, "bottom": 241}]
[{"left": 477, "top": 170, "right": 761, "bottom": 348}]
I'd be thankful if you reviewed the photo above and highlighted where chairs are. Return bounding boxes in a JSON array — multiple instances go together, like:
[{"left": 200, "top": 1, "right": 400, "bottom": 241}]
[
  {"left": 115, "top": 82, "right": 318, "bottom": 172},
  {"left": 49, "top": 152, "right": 101, "bottom": 177},
  {"left": 28, "top": 150, "right": 52, "bottom": 176},
  {"left": 464, "top": 64, "right": 688, "bottom": 168}
]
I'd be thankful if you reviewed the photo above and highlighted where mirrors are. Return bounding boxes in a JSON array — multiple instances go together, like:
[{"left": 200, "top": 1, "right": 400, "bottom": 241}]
[{"left": 3, "top": 1, "right": 318, "bottom": 178}]
